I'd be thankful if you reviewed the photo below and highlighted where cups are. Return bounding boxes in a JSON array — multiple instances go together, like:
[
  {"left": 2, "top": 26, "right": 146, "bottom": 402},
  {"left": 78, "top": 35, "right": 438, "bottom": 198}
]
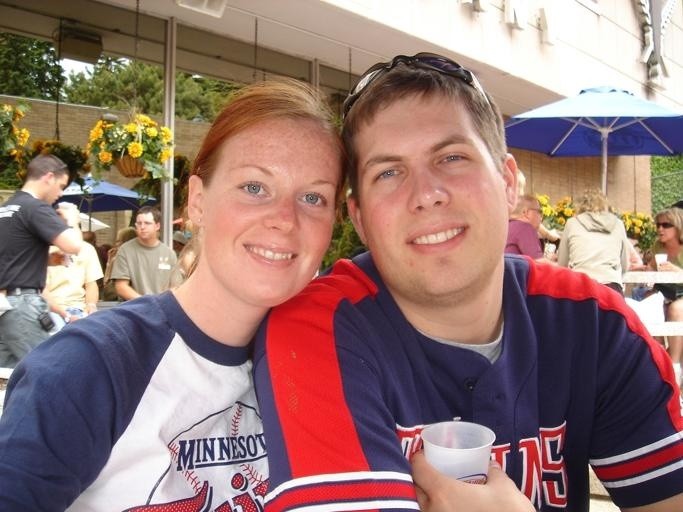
[
  {"left": 419, "top": 420, "right": 496, "bottom": 485},
  {"left": 543, "top": 243, "right": 556, "bottom": 259},
  {"left": 655, "top": 253, "right": 667, "bottom": 270}
]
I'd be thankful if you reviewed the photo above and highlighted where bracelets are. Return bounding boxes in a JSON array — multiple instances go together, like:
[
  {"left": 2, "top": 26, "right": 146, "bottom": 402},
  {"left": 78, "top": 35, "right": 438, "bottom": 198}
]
[
  {"left": 65, "top": 314, "right": 72, "bottom": 323},
  {"left": 87, "top": 303, "right": 96, "bottom": 307}
]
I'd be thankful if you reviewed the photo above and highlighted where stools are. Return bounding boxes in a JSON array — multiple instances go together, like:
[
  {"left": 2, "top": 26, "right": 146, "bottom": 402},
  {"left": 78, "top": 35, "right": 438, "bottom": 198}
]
[{"left": 640, "top": 320, "right": 682, "bottom": 337}]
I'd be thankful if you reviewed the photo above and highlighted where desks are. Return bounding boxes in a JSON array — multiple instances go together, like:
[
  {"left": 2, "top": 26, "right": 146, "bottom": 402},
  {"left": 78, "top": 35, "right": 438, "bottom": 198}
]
[{"left": 607, "top": 270, "right": 683, "bottom": 285}]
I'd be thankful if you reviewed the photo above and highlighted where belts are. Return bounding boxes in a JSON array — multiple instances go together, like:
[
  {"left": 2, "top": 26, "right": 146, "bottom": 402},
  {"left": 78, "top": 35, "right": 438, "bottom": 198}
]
[{"left": 8, "top": 288, "right": 36, "bottom": 297}]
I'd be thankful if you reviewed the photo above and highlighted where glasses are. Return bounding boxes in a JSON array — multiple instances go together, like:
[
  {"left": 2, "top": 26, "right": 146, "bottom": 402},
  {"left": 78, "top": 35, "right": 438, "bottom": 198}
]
[
  {"left": 341, "top": 51, "right": 487, "bottom": 116},
  {"left": 656, "top": 222, "right": 673, "bottom": 228}
]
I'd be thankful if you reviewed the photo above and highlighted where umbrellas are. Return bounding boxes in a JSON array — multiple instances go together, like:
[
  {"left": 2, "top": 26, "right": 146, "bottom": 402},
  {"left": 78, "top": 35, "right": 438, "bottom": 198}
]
[
  {"left": 52, "top": 175, "right": 157, "bottom": 231},
  {"left": 503, "top": 84, "right": 682, "bottom": 192}
]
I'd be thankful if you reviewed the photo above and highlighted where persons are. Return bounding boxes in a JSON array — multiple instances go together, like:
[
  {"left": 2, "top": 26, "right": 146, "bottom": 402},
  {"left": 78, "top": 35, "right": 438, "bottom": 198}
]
[
  {"left": 83, "top": 206, "right": 193, "bottom": 301},
  {"left": 1, "top": 76, "right": 348, "bottom": 512},
  {"left": 252, "top": 53, "right": 683, "bottom": 512},
  {"left": 39, "top": 201, "right": 104, "bottom": 337},
  {"left": 503, "top": 168, "right": 682, "bottom": 389},
  {"left": 1, "top": 153, "right": 83, "bottom": 368}
]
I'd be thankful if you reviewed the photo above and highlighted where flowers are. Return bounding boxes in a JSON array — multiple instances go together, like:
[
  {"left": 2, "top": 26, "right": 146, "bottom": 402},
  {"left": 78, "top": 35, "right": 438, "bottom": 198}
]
[
  {"left": 0, "top": 2, "right": 363, "bottom": 305},
  {"left": 536, "top": 193, "right": 648, "bottom": 240}
]
